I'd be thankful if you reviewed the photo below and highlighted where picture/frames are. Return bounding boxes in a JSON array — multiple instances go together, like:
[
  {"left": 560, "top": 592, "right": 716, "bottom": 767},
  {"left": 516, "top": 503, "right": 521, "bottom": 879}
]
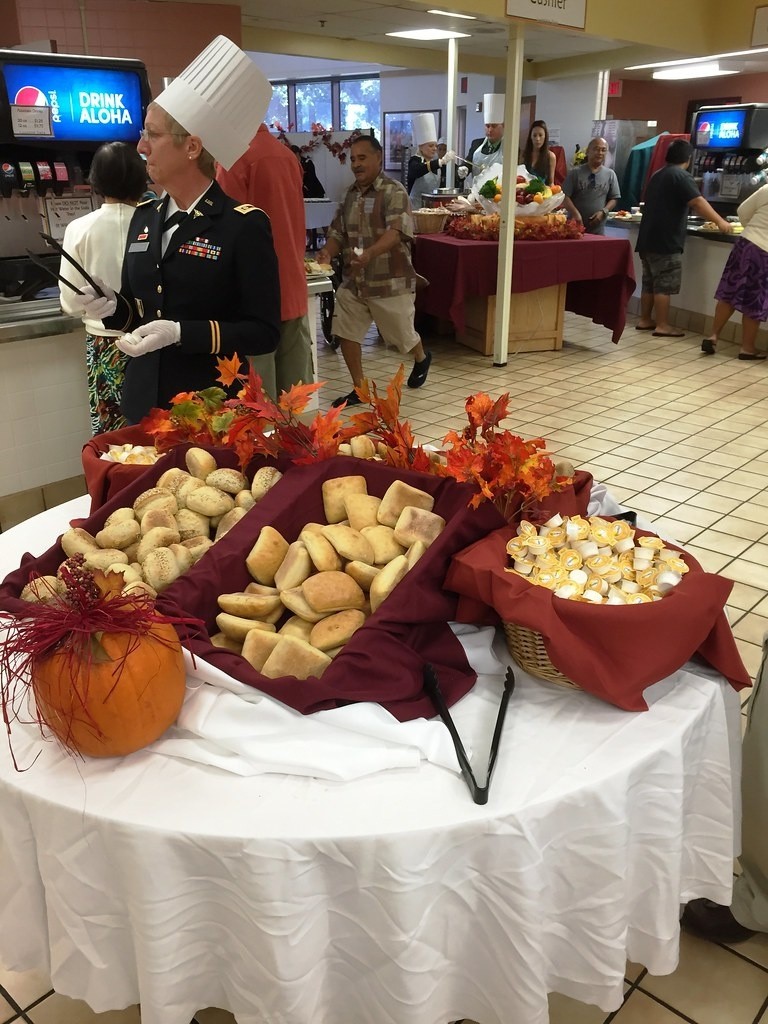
[{"left": 383, "top": 109, "right": 442, "bottom": 171}]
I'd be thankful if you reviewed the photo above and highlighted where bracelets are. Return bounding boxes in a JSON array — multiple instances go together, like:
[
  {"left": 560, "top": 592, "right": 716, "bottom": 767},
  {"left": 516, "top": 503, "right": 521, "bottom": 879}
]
[{"left": 602, "top": 208, "right": 609, "bottom": 217}]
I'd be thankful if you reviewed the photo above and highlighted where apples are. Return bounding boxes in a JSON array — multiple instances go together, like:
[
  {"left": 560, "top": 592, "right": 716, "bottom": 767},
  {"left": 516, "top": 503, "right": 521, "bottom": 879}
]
[{"left": 516, "top": 175, "right": 534, "bottom": 204}]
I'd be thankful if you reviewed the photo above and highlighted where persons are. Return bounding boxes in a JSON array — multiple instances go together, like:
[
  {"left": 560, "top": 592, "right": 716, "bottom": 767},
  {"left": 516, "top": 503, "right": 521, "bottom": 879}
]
[
  {"left": 437, "top": 137, "right": 460, "bottom": 188},
  {"left": 457, "top": 94, "right": 506, "bottom": 188},
  {"left": 701, "top": 184, "right": 768, "bottom": 360},
  {"left": 406, "top": 113, "right": 456, "bottom": 211},
  {"left": 58, "top": 141, "right": 148, "bottom": 437},
  {"left": 213, "top": 124, "right": 315, "bottom": 432},
  {"left": 290, "top": 145, "right": 325, "bottom": 251},
  {"left": 682, "top": 636, "right": 768, "bottom": 943},
  {"left": 315, "top": 135, "right": 432, "bottom": 408},
  {"left": 73, "top": 35, "right": 282, "bottom": 427},
  {"left": 561, "top": 138, "right": 621, "bottom": 235},
  {"left": 519, "top": 120, "right": 556, "bottom": 188},
  {"left": 634, "top": 139, "right": 733, "bottom": 337}
]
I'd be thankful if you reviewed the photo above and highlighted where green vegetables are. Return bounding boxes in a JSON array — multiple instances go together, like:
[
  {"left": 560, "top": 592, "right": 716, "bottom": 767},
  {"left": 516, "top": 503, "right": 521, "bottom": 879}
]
[
  {"left": 478, "top": 175, "right": 500, "bottom": 199},
  {"left": 526, "top": 176, "right": 544, "bottom": 193}
]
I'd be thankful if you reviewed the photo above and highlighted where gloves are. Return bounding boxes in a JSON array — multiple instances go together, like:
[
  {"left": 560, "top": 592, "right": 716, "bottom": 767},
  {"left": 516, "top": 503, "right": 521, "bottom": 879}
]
[
  {"left": 116, "top": 320, "right": 181, "bottom": 357},
  {"left": 458, "top": 166, "right": 468, "bottom": 179},
  {"left": 438, "top": 150, "right": 458, "bottom": 167},
  {"left": 75, "top": 275, "right": 117, "bottom": 319}
]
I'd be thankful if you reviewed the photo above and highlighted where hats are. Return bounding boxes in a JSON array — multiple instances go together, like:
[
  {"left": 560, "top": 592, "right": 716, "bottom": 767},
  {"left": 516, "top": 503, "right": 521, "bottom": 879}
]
[
  {"left": 483, "top": 93, "right": 505, "bottom": 124},
  {"left": 153, "top": 35, "right": 273, "bottom": 171},
  {"left": 438, "top": 136, "right": 447, "bottom": 146},
  {"left": 412, "top": 113, "right": 438, "bottom": 145}
]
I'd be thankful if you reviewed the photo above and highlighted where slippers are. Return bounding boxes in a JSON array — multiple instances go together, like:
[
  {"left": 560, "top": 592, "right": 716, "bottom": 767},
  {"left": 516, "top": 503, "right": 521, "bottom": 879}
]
[
  {"left": 636, "top": 324, "right": 656, "bottom": 330},
  {"left": 652, "top": 327, "right": 684, "bottom": 336},
  {"left": 739, "top": 350, "right": 767, "bottom": 359},
  {"left": 332, "top": 390, "right": 365, "bottom": 409},
  {"left": 702, "top": 339, "right": 716, "bottom": 354},
  {"left": 408, "top": 351, "right": 432, "bottom": 388}
]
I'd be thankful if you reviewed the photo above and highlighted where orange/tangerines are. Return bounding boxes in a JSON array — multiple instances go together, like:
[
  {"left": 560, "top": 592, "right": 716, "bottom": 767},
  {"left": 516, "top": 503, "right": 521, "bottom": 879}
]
[
  {"left": 494, "top": 184, "right": 502, "bottom": 203},
  {"left": 534, "top": 195, "right": 544, "bottom": 204},
  {"left": 551, "top": 185, "right": 561, "bottom": 194}
]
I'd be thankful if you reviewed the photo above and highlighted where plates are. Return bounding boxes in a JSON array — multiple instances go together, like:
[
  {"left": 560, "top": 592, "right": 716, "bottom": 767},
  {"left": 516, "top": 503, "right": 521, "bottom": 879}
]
[{"left": 608, "top": 212, "right": 618, "bottom": 217}]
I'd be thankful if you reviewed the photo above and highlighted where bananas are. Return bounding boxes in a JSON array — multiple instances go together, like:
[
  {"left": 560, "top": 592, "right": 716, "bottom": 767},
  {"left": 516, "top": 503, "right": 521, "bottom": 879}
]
[{"left": 537, "top": 185, "right": 552, "bottom": 198}]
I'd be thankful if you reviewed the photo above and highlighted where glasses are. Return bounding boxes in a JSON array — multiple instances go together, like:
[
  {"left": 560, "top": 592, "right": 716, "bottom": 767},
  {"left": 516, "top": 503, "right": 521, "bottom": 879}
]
[
  {"left": 591, "top": 174, "right": 595, "bottom": 186},
  {"left": 139, "top": 129, "right": 190, "bottom": 141}
]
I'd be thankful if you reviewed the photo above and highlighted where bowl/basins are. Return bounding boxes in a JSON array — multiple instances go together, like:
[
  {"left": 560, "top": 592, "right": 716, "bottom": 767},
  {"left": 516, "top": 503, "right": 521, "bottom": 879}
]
[{"left": 464, "top": 189, "right": 471, "bottom": 194}]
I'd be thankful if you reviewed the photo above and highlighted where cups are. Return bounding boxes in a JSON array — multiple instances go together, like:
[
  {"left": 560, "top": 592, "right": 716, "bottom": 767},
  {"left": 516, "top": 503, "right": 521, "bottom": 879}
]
[
  {"left": 631, "top": 207, "right": 640, "bottom": 214},
  {"left": 751, "top": 171, "right": 766, "bottom": 185},
  {"left": 756, "top": 152, "right": 768, "bottom": 165}
]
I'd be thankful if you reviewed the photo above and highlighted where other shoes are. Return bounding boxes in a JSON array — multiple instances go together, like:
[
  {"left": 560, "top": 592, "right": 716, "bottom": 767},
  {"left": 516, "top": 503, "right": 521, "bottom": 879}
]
[{"left": 685, "top": 898, "right": 748, "bottom": 937}]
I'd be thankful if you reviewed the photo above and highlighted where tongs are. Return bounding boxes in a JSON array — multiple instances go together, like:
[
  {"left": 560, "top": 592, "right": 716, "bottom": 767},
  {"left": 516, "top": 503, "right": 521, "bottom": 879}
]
[
  {"left": 422, "top": 662, "right": 515, "bottom": 805},
  {"left": 25, "top": 231, "right": 109, "bottom": 302}
]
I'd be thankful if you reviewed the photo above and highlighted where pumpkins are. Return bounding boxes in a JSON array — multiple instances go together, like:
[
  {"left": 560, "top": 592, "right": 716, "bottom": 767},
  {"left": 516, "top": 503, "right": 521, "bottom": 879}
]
[{"left": 32, "top": 603, "right": 184, "bottom": 757}]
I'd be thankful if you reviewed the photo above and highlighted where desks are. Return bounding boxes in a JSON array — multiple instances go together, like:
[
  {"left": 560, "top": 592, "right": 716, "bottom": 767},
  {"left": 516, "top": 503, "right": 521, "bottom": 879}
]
[
  {"left": 304, "top": 202, "right": 340, "bottom": 251},
  {"left": 0, "top": 494, "right": 743, "bottom": 1023},
  {"left": 410, "top": 231, "right": 635, "bottom": 355},
  {"left": 303, "top": 277, "right": 334, "bottom": 413}
]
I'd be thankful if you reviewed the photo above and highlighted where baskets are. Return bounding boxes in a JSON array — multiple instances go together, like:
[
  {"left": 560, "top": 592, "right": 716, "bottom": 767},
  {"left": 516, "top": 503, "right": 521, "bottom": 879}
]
[
  {"left": 412, "top": 213, "right": 450, "bottom": 234},
  {"left": 502, "top": 613, "right": 583, "bottom": 692}
]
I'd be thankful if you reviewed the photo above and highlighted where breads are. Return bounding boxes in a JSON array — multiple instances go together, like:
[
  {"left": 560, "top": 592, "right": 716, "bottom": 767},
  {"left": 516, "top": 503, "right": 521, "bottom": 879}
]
[{"left": 20, "top": 435, "right": 447, "bottom": 681}]
